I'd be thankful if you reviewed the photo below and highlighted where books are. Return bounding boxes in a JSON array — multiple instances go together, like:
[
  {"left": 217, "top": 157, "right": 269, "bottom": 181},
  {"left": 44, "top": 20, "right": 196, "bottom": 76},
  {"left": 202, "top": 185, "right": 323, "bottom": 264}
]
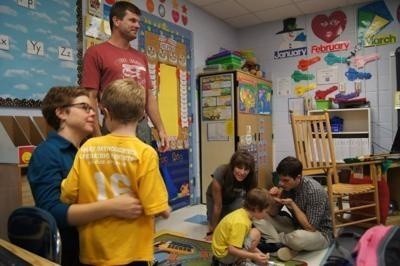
[
  {"left": 203, "top": 49, "right": 243, "bottom": 71},
  {"left": 304, "top": 99, "right": 332, "bottom": 111},
  {"left": 318, "top": 124, "right": 344, "bottom": 132}
]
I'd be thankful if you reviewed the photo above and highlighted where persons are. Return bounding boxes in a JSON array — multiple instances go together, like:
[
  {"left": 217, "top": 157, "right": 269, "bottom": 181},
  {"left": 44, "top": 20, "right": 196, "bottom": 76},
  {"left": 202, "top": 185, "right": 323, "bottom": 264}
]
[
  {"left": 208, "top": 186, "right": 273, "bottom": 265},
  {"left": 252, "top": 155, "right": 337, "bottom": 263},
  {"left": 59, "top": 77, "right": 170, "bottom": 266},
  {"left": 27, "top": 84, "right": 172, "bottom": 266},
  {"left": 203, "top": 147, "right": 259, "bottom": 241},
  {"left": 81, "top": 1, "right": 174, "bottom": 168}
]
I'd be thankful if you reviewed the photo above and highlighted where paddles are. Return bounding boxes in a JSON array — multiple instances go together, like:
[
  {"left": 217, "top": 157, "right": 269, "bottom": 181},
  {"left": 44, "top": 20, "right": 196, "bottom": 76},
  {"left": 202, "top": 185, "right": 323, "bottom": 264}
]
[
  {"left": 153, "top": 230, "right": 306, "bottom": 266},
  {"left": 185, "top": 214, "right": 208, "bottom": 226}
]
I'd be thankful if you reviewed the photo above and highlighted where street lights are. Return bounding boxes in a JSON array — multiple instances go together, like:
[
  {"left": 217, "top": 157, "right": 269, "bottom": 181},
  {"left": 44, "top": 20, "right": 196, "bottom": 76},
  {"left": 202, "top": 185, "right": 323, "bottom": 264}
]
[{"left": 335, "top": 225, "right": 400, "bottom": 266}]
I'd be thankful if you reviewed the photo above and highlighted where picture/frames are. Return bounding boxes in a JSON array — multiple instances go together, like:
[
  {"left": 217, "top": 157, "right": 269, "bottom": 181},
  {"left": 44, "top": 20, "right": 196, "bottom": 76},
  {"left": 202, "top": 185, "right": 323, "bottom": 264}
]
[{"left": 345, "top": 152, "right": 400, "bottom": 226}]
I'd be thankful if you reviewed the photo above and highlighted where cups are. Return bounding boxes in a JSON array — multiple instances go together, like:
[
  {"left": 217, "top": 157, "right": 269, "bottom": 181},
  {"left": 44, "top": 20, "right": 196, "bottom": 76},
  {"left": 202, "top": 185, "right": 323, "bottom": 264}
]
[{"left": 64, "top": 103, "right": 92, "bottom": 112}]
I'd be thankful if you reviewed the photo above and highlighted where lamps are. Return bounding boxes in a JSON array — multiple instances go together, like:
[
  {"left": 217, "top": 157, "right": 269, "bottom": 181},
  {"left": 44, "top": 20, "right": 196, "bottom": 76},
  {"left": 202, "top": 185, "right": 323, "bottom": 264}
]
[{"left": 287, "top": 110, "right": 381, "bottom": 236}]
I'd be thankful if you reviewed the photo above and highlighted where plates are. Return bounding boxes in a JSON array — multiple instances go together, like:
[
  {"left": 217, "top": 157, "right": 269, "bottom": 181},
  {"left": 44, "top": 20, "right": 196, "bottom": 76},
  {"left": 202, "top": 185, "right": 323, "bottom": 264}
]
[
  {"left": 196, "top": 68, "right": 273, "bottom": 205},
  {"left": 1, "top": 163, "right": 43, "bottom": 241},
  {"left": 306, "top": 109, "right": 373, "bottom": 165}
]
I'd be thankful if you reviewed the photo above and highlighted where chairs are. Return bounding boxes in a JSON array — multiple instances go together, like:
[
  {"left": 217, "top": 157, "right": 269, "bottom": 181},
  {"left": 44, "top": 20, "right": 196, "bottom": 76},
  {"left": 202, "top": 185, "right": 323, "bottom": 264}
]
[{"left": 278, "top": 247, "right": 297, "bottom": 261}]
